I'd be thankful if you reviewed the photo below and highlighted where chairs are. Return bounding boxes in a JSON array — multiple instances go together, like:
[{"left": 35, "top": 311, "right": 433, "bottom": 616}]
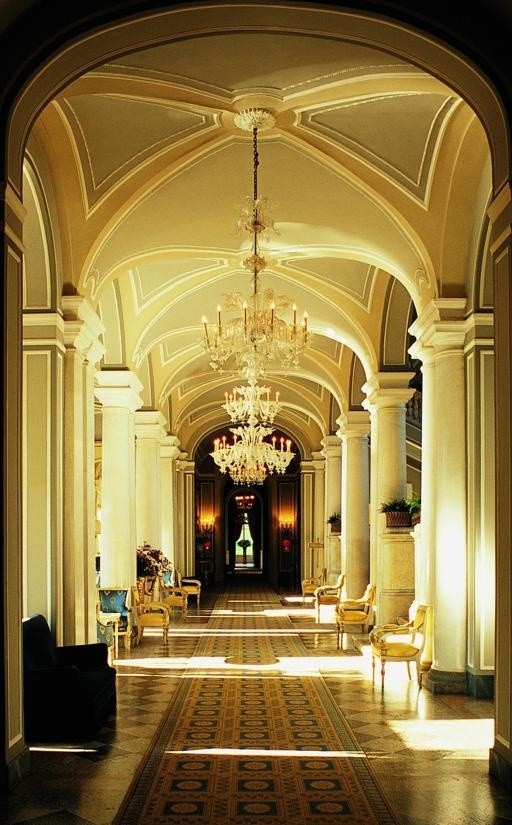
[
  {"left": 97, "top": 587, "right": 131, "bottom": 651},
  {"left": 302, "top": 568, "right": 326, "bottom": 602},
  {"left": 337, "top": 585, "right": 375, "bottom": 646},
  {"left": 132, "top": 584, "right": 170, "bottom": 646},
  {"left": 22, "top": 612, "right": 117, "bottom": 741},
  {"left": 316, "top": 575, "right": 344, "bottom": 617},
  {"left": 175, "top": 569, "right": 201, "bottom": 604},
  {"left": 160, "top": 574, "right": 188, "bottom": 621},
  {"left": 371, "top": 600, "right": 426, "bottom": 697}
]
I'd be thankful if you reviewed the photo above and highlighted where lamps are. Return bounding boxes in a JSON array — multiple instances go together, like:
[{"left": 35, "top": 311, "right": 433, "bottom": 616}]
[
  {"left": 235, "top": 494, "right": 256, "bottom": 513},
  {"left": 202, "top": 111, "right": 310, "bottom": 382},
  {"left": 212, "top": 429, "right": 295, "bottom": 486},
  {"left": 222, "top": 381, "right": 280, "bottom": 434}
]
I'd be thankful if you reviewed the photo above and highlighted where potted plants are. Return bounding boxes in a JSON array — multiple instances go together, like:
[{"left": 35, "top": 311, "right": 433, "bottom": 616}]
[
  {"left": 327, "top": 513, "right": 341, "bottom": 531},
  {"left": 380, "top": 497, "right": 412, "bottom": 527}
]
